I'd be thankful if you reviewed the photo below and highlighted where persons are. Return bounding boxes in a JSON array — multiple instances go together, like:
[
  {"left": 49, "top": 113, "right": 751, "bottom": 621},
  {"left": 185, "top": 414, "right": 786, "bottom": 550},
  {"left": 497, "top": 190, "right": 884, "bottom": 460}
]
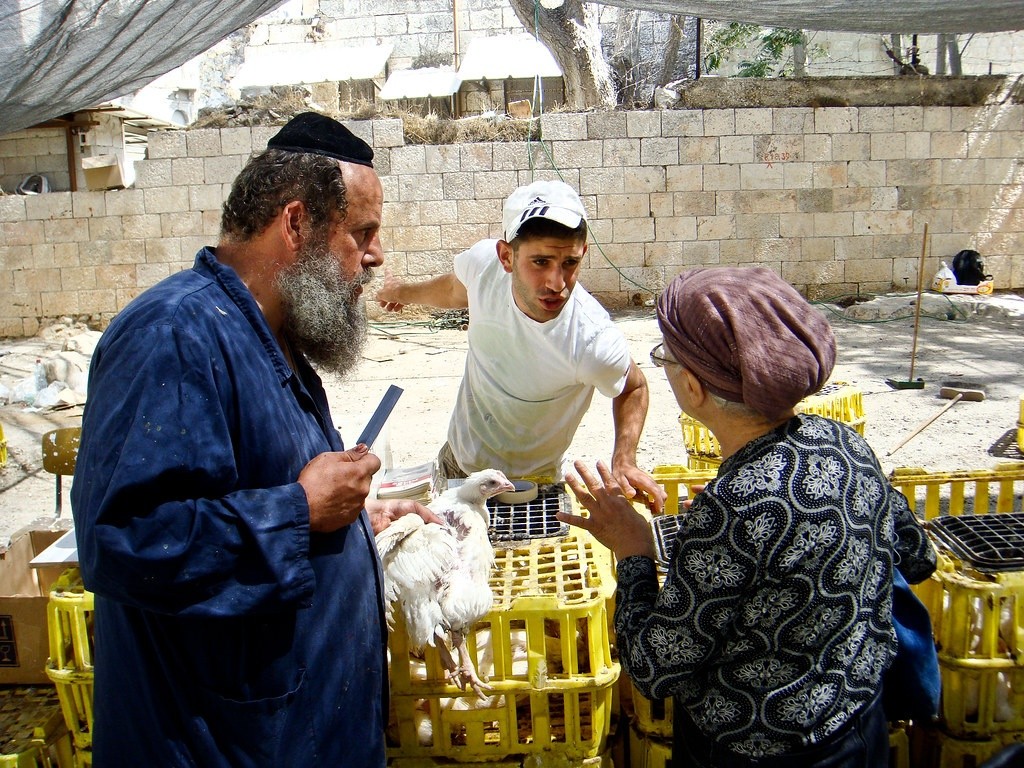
[
  {"left": 374, "top": 181, "right": 668, "bottom": 514},
  {"left": 555, "top": 263, "right": 936, "bottom": 768},
  {"left": 70, "top": 110, "right": 444, "bottom": 768}
]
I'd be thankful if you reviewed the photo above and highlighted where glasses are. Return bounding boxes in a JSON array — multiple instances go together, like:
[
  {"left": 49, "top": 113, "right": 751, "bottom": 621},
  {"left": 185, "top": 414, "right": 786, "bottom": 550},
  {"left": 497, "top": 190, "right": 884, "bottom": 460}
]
[{"left": 650, "top": 342, "right": 681, "bottom": 367}]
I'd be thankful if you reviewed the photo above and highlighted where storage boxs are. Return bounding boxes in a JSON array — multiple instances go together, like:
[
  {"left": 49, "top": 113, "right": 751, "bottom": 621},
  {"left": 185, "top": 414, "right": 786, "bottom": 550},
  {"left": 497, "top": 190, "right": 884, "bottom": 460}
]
[
  {"left": 0, "top": 383, "right": 1024, "bottom": 768},
  {"left": 80, "top": 154, "right": 135, "bottom": 192}
]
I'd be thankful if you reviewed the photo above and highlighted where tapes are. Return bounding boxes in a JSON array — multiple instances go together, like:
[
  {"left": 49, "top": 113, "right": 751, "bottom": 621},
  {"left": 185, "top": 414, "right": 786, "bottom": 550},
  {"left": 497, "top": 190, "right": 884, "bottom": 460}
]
[{"left": 498, "top": 479, "right": 538, "bottom": 503}]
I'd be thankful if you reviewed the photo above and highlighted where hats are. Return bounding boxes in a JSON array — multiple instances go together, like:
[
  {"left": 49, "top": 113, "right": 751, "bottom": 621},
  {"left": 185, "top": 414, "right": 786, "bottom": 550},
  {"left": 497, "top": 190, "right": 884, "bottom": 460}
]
[
  {"left": 267, "top": 112, "right": 375, "bottom": 169},
  {"left": 655, "top": 266, "right": 837, "bottom": 418},
  {"left": 502, "top": 180, "right": 588, "bottom": 243}
]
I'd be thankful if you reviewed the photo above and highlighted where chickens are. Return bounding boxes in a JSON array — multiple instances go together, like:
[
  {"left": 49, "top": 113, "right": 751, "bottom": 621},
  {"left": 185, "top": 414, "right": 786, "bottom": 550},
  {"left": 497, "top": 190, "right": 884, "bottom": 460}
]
[
  {"left": 940, "top": 587, "right": 1024, "bottom": 726},
  {"left": 370, "top": 467, "right": 516, "bottom": 700},
  {"left": 379, "top": 625, "right": 564, "bottom": 746}
]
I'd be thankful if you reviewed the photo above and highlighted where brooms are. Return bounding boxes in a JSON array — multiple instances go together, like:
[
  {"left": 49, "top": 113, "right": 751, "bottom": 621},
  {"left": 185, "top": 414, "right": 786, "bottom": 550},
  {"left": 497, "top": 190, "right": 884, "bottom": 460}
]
[{"left": 885, "top": 381, "right": 987, "bottom": 456}]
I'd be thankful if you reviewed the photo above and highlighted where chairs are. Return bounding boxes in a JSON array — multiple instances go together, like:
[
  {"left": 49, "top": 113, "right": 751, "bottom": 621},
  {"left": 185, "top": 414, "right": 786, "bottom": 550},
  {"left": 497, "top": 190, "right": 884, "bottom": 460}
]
[{"left": 8, "top": 426, "right": 82, "bottom": 543}]
